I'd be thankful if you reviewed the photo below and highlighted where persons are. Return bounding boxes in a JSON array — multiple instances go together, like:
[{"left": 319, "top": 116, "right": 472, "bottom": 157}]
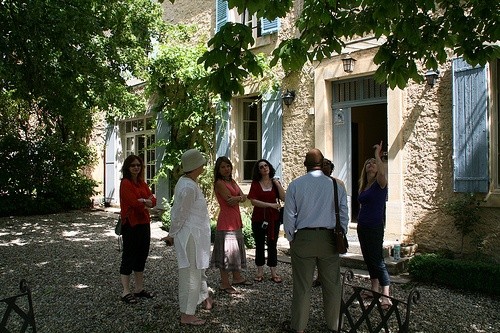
[
  {"left": 211, "top": 155, "right": 252, "bottom": 294},
  {"left": 160, "top": 149, "right": 214, "bottom": 326},
  {"left": 282, "top": 148, "right": 348, "bottom": 333},
  {"left": 312, "top": 158, "right": 346, "bottom": 287},
  {"left": 119, "top": 154, "right": 157, "bottom": 305},
  {"left": 247, "top": 159, "right": 286, "bottom": 283},
  {"left": 356, "top": 141, "right": 393, "bottom": 310}
]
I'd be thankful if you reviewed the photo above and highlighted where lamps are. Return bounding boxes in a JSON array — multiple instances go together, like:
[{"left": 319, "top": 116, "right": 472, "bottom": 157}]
[
  {"left": 424, "top": 68, "right": 440, "bottom": 88},
  {"left": 341, "top": 54, "right": 357, "bottom": 72},
  {"left": 282, "top": 89, "right": 293, "bottom": 108}
]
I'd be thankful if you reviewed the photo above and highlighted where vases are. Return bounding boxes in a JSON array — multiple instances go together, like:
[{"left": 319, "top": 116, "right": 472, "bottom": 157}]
[{"left": 400, "top": 244, "right": 418, "bottom": 257}]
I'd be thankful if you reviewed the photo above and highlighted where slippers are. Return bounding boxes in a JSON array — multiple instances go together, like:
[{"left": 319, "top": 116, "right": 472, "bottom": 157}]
[
  {"left": 360, "top": 290, "right": 382, "bottom": 299},
  {"left": 121, "top": 293, "right": 138, "bottom": 304},
  {"left": 219, "top": 285, "right": 238, "bottom": 294},
  {"left": 253, "top": 276, "right": 264, "bottom": 282},
  {"left": 381, "top": 298, "right": 393, "bottom": 310},
  {"left": 231, "top": 280, "right": 253, "bottom": 286},
  {"left": 205, "top": 298, "right": 216, "bottom": 310},
  {"left": 181, "top": 313, "right": 206, "bottom": 325},
  {"left": 134, "top": 289, "right": 155, "bottom": 299},
  {"left": 271, "top": 276, "right": 283, "bottom": 283}
]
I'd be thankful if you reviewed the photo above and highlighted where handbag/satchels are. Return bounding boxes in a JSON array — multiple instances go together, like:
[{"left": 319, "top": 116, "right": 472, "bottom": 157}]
[
  {"left": 115, "top": 216, "right": 124, "bottom": 236},
  {"left": 278, "top": 207, "right": 284, "bottom": 224},
  {"left": 333, "top": 225, "right": 348, "bottom": 254}
]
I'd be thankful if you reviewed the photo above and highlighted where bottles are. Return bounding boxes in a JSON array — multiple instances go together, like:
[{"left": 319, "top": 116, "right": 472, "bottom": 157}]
[{"left": 394, "top": 239, "right": 401, "bottom": 261}]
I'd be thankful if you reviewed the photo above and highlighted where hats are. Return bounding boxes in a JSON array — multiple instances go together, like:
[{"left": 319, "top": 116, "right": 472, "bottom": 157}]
[{"left": 181, "top": 149, "right": 206, "bottom": 172}]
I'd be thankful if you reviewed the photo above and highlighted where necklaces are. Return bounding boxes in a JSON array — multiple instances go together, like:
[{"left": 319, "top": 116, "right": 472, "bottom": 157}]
[{"left": 262, "top": 179, "right": 270, "bottom": 190}]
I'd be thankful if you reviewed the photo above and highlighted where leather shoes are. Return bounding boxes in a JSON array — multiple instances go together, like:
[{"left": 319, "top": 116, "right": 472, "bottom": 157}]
[{"left": 282, "top": 320, "right": 304, "bottom": 333}]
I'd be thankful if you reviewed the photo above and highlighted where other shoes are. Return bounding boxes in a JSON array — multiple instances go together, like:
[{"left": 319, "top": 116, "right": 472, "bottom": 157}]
[{"left": 312, "top": 280, "right": 320, "bottom": 287}]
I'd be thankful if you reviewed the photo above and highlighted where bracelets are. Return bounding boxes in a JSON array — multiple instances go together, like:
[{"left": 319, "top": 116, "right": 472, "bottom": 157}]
[{"left": 142, "top": 199, "right": 146, "bottom": 204}]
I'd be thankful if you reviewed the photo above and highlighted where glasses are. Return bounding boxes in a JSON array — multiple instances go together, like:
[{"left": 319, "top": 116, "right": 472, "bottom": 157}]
[
  {"left": 260, "top": 164, "right": 268, "bottom": 169},
  {"left": 130, "top": 164, "right": 142, "bottom": 167}
]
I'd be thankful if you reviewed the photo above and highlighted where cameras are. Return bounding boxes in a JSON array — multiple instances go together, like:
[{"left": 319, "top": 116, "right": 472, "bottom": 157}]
[{"left": 262, "top": 221, "right": 268, "bottom": 229}]
